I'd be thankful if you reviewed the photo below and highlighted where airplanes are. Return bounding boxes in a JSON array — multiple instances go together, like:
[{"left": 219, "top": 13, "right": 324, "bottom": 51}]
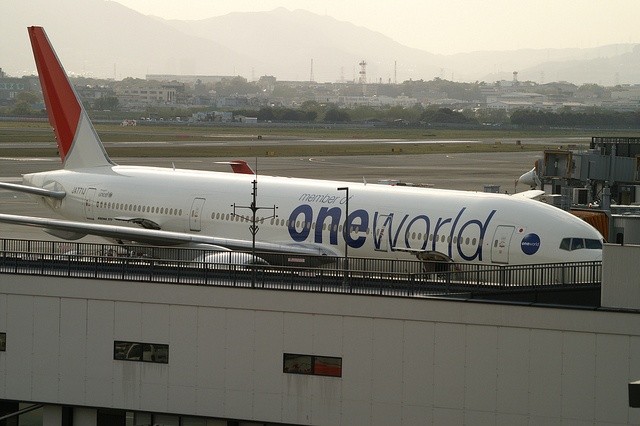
[{"left": 0, "top": 26, "right": 608, "bottom": 304}]
